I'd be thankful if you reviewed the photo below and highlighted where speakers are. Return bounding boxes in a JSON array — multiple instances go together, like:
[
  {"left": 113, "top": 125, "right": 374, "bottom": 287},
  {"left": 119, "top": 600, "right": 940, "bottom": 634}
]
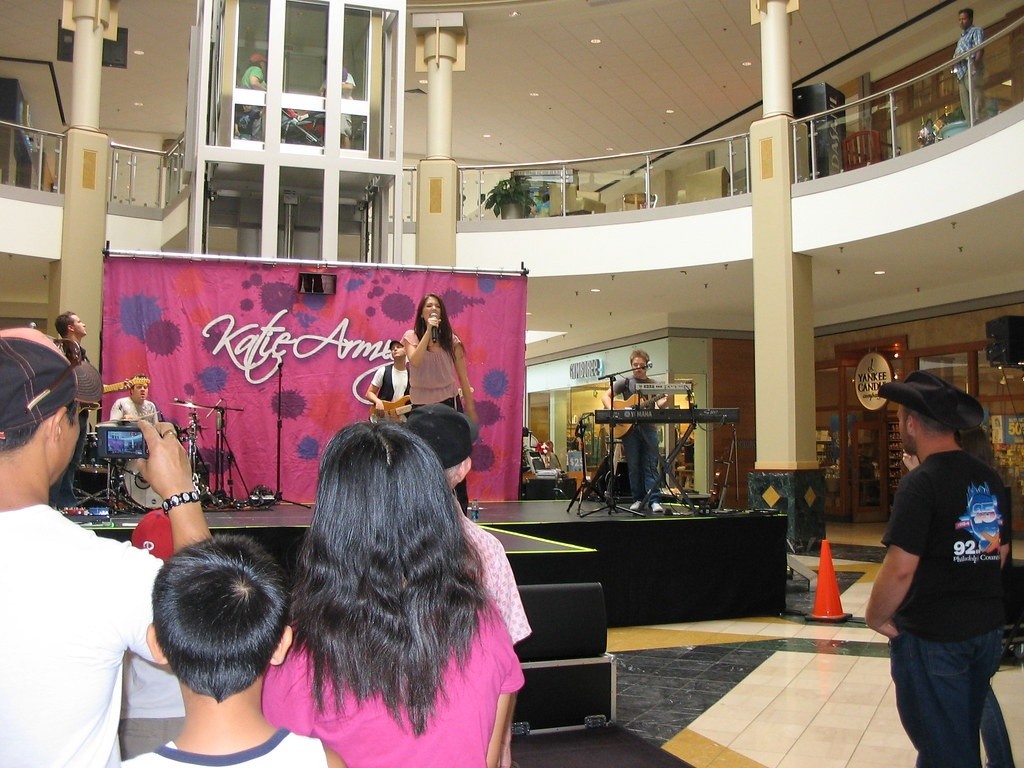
[
  {"left": 986, "top": 317, "right": 1024, "bottom": 363},
  {"left": 511, "top": 581, "right": 609, "bottom": 664}
]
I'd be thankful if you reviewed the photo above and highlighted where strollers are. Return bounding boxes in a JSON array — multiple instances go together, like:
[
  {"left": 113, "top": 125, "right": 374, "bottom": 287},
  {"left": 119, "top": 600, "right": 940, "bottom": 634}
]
[{"left": 281, "top": 107, "right": 325, "bottom": 148}]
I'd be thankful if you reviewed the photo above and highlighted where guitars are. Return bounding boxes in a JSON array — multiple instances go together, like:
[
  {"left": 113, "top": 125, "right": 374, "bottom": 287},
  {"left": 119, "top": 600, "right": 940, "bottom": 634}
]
[
  {"left": 74, "top": 376, "right": 151, "bottom": 414},
  {"left": 368, "top": 385, "right": 476, "bottom": 425},
  {"left": 602, "top": 379, "right": 697, "bottom": 439}
]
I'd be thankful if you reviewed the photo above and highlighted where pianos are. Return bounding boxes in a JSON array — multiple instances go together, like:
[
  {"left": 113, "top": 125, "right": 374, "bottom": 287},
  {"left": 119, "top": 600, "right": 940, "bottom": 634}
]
[{"left": 594, "top": 407, "right": 740, "bottom": 516}]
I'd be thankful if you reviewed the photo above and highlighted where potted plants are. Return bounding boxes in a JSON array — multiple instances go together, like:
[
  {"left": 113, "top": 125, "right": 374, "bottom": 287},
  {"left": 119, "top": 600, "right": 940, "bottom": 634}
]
[
  {"left": 485, "top": 174, "right": 535, "bottom": 218},
  {"left": 941, "top": 106, "right": 967, "bottom": 139}
]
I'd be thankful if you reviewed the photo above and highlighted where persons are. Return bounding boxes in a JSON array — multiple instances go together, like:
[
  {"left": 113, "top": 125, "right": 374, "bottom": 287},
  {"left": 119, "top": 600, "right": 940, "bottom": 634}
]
[
  {"left": 261, "top": 404, "right": 534, "bottom": 768},
  {"left": 1, "top": 325, "right": 222, "bottom": 768},
  {"left": 320, "top": 59, "right": 356, "bottom": 149},
  {"left": 239, "top": 53, "right": 267, "bottom": 90},
  {"left": 602, "top": 349, "right": 669, "bottom": 512},
  {"left": 876, "top": 370, "right": 1015, "bottom": 768},
  {"left": 365, "top": 339, "right": 409, "bottom": 425},
  {"left": 399, "top": 292, "right": 480, "bottom": 518},
  {"left": 111, "top": 534, "right": 346, "bottom": 768},
  {"left": 109, "top": 374, "right": 159, "bottom": 467},
  {"left": 234, "top": 105, "right": 262, "bottom": 141},
  {"left": 49, "top": 310, "right": 89, "bottom": 507}
]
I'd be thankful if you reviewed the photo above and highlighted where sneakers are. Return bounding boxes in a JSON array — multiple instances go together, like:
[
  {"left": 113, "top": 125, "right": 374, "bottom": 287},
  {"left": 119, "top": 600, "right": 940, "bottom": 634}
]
[
  {"left": 629, "top": 502, "right": 641, "bottom": 510},
  {"left": 652, "top": 503, "right": 662, "bottom": 512}
]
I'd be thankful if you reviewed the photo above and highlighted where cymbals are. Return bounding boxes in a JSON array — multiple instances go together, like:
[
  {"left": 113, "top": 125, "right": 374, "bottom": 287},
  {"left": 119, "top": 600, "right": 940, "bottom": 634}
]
[{"left": 169, "top": 402, "right": 209, "bottom": 409}]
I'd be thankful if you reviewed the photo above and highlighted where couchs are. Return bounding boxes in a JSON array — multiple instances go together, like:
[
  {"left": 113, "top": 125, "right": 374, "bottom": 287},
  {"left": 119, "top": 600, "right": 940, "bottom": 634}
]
[{"left": 547, "top": 182, "right": 607, "bottom": 216}]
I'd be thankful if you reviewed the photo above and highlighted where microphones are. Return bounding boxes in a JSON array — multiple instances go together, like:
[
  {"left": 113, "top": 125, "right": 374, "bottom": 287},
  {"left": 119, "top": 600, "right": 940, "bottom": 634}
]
[
  {"left": 174, "top": 397, "right": 186, "bottom": 403},
  {"left": 205, "top": 399, "right": 223, "bottom": 418},
  {"left": 647, "top": 362, "right": 653, "bottom": 368},
  {"left": 430, "top": 312, "right": 438, "bottom": 343}
]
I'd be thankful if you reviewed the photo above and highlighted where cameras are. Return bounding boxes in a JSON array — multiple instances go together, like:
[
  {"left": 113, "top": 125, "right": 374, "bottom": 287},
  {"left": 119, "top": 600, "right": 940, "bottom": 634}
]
[{"left": 96, "top": 422, "right": 151, "bottom": 461}]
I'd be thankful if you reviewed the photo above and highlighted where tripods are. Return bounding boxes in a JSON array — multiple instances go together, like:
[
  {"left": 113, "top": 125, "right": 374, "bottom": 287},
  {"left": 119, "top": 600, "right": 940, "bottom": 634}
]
[
  {"left": 208, "top": 362, "right": 312, "bottom": 509},
  {"left": 567, "top": 364, "right": 646, "bottom": 518},
  {"left": 77, "top": 458, "right": 146, "bottom": 514}
]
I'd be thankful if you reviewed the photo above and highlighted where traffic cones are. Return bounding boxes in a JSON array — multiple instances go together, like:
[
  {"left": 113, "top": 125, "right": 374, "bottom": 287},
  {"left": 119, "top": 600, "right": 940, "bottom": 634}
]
[{"left": 805, "top": 539, "right": 853, "bottom": 624}]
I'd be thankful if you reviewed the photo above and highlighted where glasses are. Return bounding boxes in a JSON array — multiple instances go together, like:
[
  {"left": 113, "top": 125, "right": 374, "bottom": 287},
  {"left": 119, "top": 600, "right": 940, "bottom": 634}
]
[{"left": 27, "top": 338, "right": 83, "bottom": 414}]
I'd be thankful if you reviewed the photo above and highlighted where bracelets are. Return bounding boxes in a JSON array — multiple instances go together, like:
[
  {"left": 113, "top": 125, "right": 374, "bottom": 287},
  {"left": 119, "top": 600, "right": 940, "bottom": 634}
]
[{"left": 161, "top": 491, "right": 201, "bottom": 515}]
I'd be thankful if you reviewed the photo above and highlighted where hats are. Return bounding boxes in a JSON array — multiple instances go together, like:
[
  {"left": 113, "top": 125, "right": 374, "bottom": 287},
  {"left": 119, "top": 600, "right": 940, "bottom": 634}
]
[
  {"left": 389, "top": 340, "right": 405, "bottom": 351},
  {"left": 0, "top": 328, "right": 100, "bottom": 432},
  {"left": 877, "top": 370, "right": 985, "bottom": 430},
  {"left": 409, "top": 403, "right": 479, "bottom": 469}
]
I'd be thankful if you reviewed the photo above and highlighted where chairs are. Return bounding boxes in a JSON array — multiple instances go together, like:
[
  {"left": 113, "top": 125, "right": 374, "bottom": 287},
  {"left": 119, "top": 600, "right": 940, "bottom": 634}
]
[{"left": 841, "top": 129, "right": 901, "bottom": 171}]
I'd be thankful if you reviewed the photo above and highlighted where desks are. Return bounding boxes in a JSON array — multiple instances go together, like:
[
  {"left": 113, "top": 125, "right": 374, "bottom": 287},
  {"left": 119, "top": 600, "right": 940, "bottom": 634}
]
[
  {"left": 622, "top": 193, "right": 652, "bottom": 211},
  {"left": 862, "top": 409, "right": 911, "bottom": 501}
]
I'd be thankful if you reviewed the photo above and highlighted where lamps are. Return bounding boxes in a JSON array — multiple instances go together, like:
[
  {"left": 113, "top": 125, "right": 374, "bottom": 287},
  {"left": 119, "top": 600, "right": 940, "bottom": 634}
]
[{"left": 1000, "top": 376, "right": 1007, "bottom": 384}]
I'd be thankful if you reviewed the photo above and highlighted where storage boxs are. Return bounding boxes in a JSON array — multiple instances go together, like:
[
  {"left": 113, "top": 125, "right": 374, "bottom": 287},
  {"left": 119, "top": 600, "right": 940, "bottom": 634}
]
[{"left": 513, "top": 652, "right": 618, "bottom": 736}]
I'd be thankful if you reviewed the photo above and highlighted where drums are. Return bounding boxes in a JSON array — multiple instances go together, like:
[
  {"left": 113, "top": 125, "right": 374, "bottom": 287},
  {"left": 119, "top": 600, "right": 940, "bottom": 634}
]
[{"left": 111, "top": 458, "right": 165, "bottom": 513}]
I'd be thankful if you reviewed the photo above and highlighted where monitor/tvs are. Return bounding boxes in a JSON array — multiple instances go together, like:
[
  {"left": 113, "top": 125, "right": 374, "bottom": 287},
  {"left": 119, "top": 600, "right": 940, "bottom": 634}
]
[{"left": 56, "top": 19, "right": 129, "bottom": 68}]
